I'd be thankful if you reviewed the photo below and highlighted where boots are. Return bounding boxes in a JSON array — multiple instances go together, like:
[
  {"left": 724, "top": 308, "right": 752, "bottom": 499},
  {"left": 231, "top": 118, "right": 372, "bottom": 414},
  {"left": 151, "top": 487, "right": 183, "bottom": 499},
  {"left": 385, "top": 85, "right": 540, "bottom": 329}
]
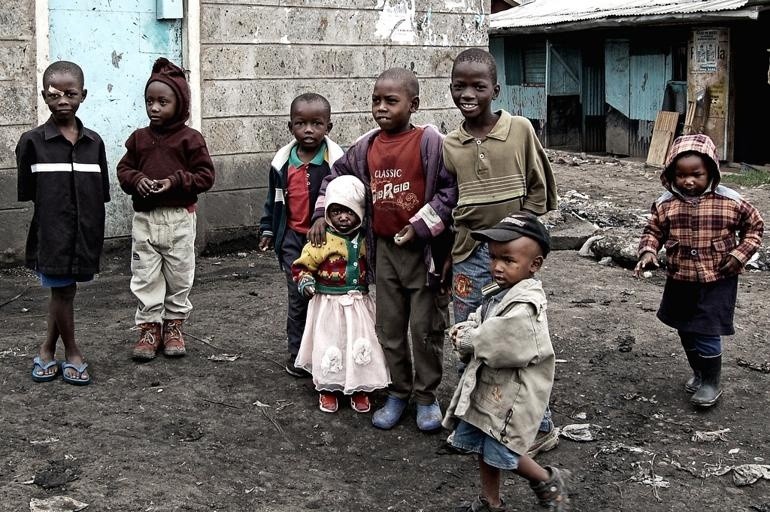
[
  {"left": 689, "top": 351, "right": 723, "bottom": 407},
  {"left": 684, "top": 348, "right": 703, "bottom": 394}
]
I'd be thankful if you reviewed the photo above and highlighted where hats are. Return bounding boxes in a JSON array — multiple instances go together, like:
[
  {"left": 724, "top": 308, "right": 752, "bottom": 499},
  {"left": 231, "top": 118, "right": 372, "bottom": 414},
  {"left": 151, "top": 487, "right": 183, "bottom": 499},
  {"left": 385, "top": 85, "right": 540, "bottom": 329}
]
[{"left": 470, "top": 211, "right": 551, "bottom": 253}]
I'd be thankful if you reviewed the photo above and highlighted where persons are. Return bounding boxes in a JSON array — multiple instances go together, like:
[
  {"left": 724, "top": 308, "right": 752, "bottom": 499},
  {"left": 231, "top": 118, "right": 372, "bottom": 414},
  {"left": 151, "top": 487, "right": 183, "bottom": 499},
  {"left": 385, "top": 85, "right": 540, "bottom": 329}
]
[
  {"left": 632, "top": 135, "right": 764, "bottom": 410},
  {"left": 306, "top": 68, "right": 456, "bottom": 431},
  {"left": 291, "top": 175, "right": 390, "bottom": 412},
  {"left": 118, "top": 57, "right": 216, "bottom": 361},
  {"left": 439, "top": 49, "right": 556, "bottom": 460},
  {"left": 258, "top": 93, "right": 345, "bottom": 378},
  {"left": 448, "top": 210, "right": 570, "bottom": 512},
  {"left": 16, "top": 62, "right": 110, "bottom": 385}
]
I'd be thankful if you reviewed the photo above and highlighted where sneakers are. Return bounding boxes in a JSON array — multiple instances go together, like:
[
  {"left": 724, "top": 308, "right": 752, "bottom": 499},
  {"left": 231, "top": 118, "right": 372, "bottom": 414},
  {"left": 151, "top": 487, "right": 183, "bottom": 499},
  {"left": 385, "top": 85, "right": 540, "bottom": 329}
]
[
  {"left": 285, "top": 354, "right": 305, "bottom": 377},
  {"left": 162, "top": 319, "right": 186, "bottom": 356},
  {"left": 529, "top": 465, "right": 572, "bottom": 512},
  {"left": 525, "top": 425, "right": 560, "bottom": 459},
  {"left": 463, "top": 494, "right": 506, "bottom": 512},
  {"left": 132, "top": 322, "right": 162, "bottom": 359}
]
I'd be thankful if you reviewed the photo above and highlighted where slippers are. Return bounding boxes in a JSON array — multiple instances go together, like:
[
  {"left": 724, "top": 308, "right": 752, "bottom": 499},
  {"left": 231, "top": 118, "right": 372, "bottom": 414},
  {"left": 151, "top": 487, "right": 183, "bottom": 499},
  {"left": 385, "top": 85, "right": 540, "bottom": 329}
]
[
  {"left": 61, "top": 361, "right": 89, "bottom": 385},
  {"left": 32, "top": 356, "right": 58, "bottom": 382}
]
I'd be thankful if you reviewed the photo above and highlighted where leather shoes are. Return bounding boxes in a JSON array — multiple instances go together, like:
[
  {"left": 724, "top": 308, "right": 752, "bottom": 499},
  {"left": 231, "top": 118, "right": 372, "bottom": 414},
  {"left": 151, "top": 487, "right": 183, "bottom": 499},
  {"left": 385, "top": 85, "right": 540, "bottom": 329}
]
[
  {"left": 371, "top": 394, "right": 407, "bottom": 430},
  {"left": 319, "top": 393, "right": 338, "bottom": 413},
  {"left": 350, "top": 393, "right": 371, "bottom": 412},
  {"left": 416, "top": 396, "right": 443, "bottom": 431}
]
